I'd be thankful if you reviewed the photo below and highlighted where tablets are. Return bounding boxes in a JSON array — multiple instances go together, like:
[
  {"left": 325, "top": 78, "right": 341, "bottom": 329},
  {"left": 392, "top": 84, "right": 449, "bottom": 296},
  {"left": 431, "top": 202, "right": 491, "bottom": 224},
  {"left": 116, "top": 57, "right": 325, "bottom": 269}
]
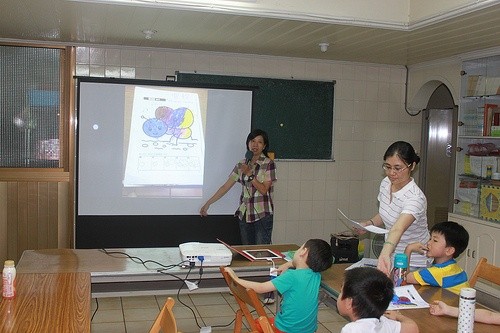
[{"left": 243, "top": 249, "right": 282, "bottom": 260}]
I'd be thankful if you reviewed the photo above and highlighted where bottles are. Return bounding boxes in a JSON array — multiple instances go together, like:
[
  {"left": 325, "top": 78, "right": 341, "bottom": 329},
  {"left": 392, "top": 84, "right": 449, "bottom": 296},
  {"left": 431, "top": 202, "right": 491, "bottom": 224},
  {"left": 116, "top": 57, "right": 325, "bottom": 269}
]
[
  {"left": 486, "top": 165, "right": 492, "bottom": 181},
  {"left": 457, "top": 288, "right": 477, "bottom": 333},
  {"left": 2, "top": 259, "right": 17, "bottom": 301},
  {"left": 393, "top": 253, "right": 408, "bottom": 287}
]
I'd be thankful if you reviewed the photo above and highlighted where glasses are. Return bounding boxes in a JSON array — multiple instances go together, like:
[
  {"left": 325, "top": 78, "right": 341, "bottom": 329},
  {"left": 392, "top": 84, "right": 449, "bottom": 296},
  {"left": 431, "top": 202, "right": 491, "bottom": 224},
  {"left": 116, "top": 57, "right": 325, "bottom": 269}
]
[{"left": 382, "top": 162, "right": 412, "bottom": 174}]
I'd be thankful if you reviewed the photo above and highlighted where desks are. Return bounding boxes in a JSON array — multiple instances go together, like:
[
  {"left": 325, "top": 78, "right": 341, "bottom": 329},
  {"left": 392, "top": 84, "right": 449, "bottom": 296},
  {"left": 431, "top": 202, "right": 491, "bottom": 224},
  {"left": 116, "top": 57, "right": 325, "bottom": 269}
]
[
  {"left": 14, "top": 244, "right": 300, "bottom": 314},
  {"left": 0, "top": 272, "right": 92, "bottom": 333},
  {"left": 320, "top": 262, "right": 500, "bottom": 333}
]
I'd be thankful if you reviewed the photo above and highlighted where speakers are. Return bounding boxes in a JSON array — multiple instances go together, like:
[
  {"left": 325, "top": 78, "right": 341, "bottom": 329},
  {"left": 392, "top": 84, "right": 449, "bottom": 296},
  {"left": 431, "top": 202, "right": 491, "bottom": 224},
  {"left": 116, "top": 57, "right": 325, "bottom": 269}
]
[{"left": 369, "top": 233, "right": 385, "bottom": 259}]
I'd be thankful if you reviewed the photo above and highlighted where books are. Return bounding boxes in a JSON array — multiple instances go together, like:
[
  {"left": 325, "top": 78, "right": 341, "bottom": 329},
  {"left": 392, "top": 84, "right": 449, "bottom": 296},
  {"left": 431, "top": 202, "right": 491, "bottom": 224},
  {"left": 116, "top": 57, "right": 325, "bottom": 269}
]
[{"left": 462, "top": 74, "right": 500, "bottom": 137}]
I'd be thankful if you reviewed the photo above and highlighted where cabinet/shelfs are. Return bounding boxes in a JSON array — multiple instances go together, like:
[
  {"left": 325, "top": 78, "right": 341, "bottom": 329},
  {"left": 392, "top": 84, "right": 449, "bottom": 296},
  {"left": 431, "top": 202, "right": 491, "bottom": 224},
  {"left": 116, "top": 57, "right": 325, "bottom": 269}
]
[
  {"left": 452, "top": 55, "right": 500, "bottom": 223},
  {"left": 448, "top": 213, "right": 500, "bottom": 298}
]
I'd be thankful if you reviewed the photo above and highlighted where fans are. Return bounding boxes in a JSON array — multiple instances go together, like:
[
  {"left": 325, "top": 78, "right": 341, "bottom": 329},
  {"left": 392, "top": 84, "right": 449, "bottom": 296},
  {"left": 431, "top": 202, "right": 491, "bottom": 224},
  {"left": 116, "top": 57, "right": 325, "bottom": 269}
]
[{"left": 370, "top": 231, "right": 386, "bottom": 259}]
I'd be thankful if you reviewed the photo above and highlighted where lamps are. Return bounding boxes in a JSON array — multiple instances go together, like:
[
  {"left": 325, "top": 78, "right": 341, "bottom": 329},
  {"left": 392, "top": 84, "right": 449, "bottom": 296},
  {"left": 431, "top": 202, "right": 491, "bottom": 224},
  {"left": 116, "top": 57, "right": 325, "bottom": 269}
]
[
  {"left": 142, "top": 29, "right": 157, "bottom": 39},
  {"left": 318, "top": 43, "right": 330, "bottom": 53}
]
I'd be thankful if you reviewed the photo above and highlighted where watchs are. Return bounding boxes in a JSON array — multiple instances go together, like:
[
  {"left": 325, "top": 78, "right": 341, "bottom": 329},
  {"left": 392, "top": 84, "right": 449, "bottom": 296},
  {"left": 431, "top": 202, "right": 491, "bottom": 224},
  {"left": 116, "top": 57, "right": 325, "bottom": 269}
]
[{"left": 248, "top": 175, "right": 254, "bottom": 182}]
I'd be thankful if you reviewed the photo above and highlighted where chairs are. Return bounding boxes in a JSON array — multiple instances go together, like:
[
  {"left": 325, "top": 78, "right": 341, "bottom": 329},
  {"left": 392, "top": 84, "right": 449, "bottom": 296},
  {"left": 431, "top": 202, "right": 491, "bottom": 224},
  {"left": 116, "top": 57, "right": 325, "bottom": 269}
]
[
  {"left": 148, "top": 296, "right": 180, "bottom": 333},
  {"left": 218, "top": 265, "right": 282, "bottom": 333},
  {"left": 469, "top": 257, "right": 500, "bottom": 288}
]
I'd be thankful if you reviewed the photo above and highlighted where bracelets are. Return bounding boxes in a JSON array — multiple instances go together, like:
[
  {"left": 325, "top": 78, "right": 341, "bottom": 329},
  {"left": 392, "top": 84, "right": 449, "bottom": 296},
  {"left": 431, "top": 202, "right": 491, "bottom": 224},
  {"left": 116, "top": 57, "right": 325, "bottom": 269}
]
[
  {"left": 383, "top": 242, "right": 394, "bottom": 245},
  {"left": 370, "top": 220, "right": 374, "bottom": 225}
]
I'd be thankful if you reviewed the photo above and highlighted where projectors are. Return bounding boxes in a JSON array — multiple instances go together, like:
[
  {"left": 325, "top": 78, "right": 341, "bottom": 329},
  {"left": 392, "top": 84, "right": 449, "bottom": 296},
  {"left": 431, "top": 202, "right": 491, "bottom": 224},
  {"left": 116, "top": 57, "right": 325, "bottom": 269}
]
[{"left": 179, "top": 241, "right": 233, "bottom": 267}]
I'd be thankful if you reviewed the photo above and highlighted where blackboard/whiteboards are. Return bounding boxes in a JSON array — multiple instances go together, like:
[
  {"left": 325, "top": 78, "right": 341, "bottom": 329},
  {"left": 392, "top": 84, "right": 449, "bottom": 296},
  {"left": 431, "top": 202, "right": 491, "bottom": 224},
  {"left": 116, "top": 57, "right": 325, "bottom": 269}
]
[{"left": 175, "top": 70, "right": 337, "bottom": 162}]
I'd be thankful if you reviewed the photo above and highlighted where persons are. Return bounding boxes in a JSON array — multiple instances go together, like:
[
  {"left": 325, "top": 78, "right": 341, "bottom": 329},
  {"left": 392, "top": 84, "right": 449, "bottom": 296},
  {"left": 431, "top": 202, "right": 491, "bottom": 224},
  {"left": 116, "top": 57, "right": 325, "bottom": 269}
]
[
  {"left": 430, "top": 301, "right": 500, "bottom": 325},
  {"left": 336, "top": 267, "right": 419, "bottom": 333},
  {"left": 404, "top": 221, "right": 470, "bottom": 296},
  {"left": 223, "top": 239, "right": 332, "bottom": 333},
  {"left": 201, "top": 129, "right": 275, "bottom": 246},
  {"left": 353, "top": 141, "right": 434, "bottom": 278}
]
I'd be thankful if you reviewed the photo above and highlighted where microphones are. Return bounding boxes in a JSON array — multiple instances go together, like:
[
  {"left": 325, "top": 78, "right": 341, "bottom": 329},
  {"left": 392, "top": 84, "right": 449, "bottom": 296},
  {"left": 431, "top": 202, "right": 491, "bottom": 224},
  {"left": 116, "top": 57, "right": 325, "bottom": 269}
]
[{"left": 244, "top": 150, "right": 254, "bottom": 165}]
